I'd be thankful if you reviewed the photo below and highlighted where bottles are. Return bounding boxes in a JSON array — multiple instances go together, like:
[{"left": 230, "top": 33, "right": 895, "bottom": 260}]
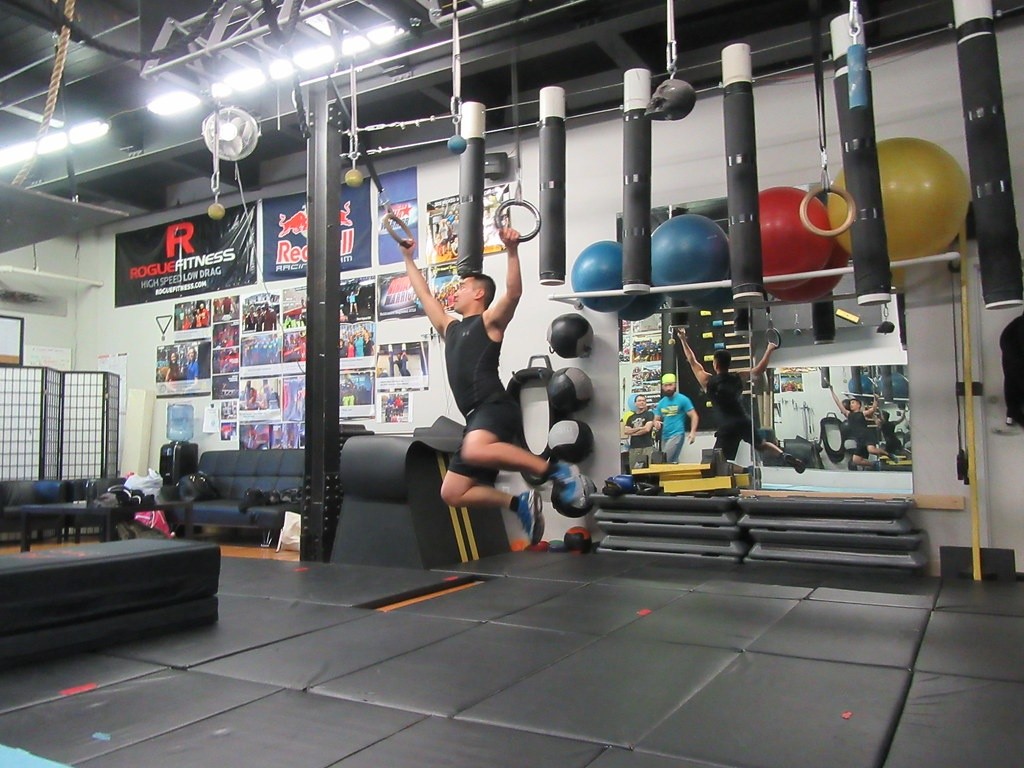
[
  {"left": 86, "top": 476, "right": 97, "bottom": 508},
  {"left": 166, "top": 403, "right": 195, "bottom": 442}
]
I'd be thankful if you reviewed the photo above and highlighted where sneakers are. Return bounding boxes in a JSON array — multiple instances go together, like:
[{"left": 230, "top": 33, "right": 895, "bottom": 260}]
[
  {"left": 515, "top": 488, "right": 545, "bottom": 545},
  {"left": 550, "top": 460, "right": 580, "bottom": 504}
]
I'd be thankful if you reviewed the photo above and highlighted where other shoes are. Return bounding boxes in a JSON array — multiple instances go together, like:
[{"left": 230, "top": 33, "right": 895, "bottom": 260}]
[
  {"left": 780, "top": 451, "right": 805, "bottom": 474},
  {"left": 888, "top": 454, "right": 898, "bottom": 463}
]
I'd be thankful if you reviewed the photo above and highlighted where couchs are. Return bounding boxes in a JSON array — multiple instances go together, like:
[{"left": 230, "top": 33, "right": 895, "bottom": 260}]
[
  {"left": 0, "top": 476, "right": 127, "bottom": 544},
  {"left": 160, "top": 449, "right": 307, "bottom": 548}
]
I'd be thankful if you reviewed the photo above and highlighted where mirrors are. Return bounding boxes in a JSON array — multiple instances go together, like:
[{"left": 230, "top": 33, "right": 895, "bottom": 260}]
[{"left": 615, "top": 183, "right": 912, "bottom": 497}]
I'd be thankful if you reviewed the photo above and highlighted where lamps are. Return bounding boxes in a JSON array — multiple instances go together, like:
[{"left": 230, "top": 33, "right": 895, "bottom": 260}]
[{"left": 0, "top": 105, "right": 147, "bottom": 169}]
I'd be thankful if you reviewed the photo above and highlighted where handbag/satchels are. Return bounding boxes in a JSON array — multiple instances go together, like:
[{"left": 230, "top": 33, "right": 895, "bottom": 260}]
[
  {"left": 124, "top": 466, "right": 163, "bottom": 504},
  {"left": 275, "top": 510, "right": 301, "bottom": 553},
  {"left": 179, "top": 471, "right": 218, "bottom": 504}
]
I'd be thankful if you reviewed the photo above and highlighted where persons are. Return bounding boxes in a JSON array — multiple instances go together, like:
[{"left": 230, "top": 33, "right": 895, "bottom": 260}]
[
  {"left": 165, "top": 284, "right": 408, "bottom": 448},
  {"left": 401, "top": 226, "right": 585, "bottom": 548},
  {"left": 623, "top": 395, "right": 655, "bottom": 473},
  {"left": 676, "top": 327, "right": 806, "bottom": 474},
  {"left": 873, "top": 409, "right": 912, "bottom": 463},
  {"left": 830, "top": 385, "right": 899, "bottom": 471},
  {"left": 652, "top": 373, "right": 699, "bottom": 462}
]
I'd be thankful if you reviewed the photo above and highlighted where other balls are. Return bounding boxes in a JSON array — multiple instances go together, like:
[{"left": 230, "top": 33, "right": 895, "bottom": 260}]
[
  {"left": 547, "top": 139, "right": 969, "bottom": 555},
  {"left": 848, "top": 372, "right": 909, "bottom": 402}
]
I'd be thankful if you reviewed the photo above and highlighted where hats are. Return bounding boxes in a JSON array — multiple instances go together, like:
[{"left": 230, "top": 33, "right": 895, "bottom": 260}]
[{"left": 662, "top": 373, "right": 676, "bottom": 384}]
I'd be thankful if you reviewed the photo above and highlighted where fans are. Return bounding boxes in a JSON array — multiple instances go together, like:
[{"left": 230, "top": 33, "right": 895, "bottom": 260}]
[{"left": 201, "top": 107, "right": 261, "bottom": 162}]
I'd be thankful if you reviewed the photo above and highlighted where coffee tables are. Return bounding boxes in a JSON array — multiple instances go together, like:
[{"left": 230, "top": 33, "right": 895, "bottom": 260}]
[{"left": 17, "top": 501, "right": 193, "bottom": 552}]
